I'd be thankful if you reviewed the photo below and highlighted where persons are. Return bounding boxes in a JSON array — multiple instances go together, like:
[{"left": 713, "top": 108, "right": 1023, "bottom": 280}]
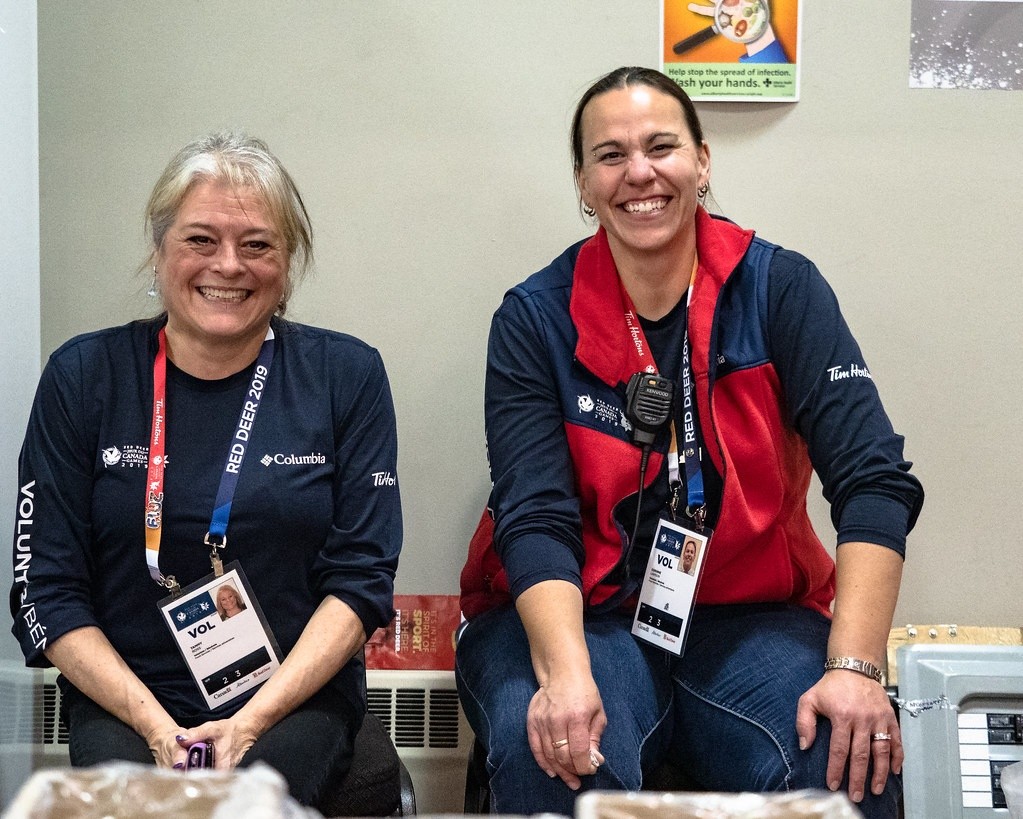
[
  {"left": 217, "top": 585, "right": 249, "bottom": 623},
  {"left": 678, "top": 541, "right": 696, "bottom": 576},
  {"left": 449, "top": 64, "right": 927, "bottom": 819},
  {"left": 9, "top": 131, "right": 417, "bottom": 818}
]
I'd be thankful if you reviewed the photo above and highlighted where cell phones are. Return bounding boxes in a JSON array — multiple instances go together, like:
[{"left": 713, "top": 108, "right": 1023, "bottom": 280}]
[{"left": 185, "top": 742, "right": 215, "bottom": 773}]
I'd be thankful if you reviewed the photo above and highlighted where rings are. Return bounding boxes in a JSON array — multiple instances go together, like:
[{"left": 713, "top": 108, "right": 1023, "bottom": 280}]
[
  {"left": 870, "top": 732, "right": 892, "bottom": 742},
  {"left": 552, "top": 738, "right": 568, "bottom": 749}
]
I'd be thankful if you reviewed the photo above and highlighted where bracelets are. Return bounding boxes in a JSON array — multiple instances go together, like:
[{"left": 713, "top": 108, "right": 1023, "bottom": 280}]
[{"left": 825, "top": 656, "right": 887, "bottom": 687}]
